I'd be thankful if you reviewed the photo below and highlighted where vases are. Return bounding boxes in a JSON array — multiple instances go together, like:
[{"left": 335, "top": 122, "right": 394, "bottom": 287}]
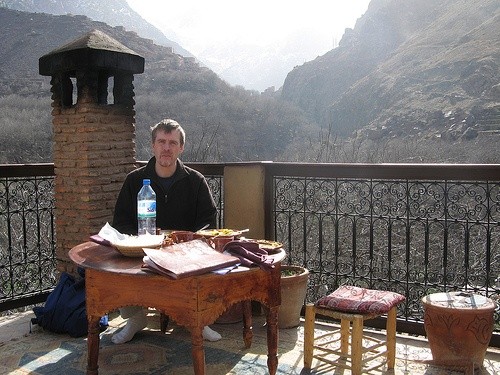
[
  {"left": 421, "top": 291, "right": 496, "bottom": 370},
  {"left": 214, "top": 298, "right": 244, "bottom": 324}
]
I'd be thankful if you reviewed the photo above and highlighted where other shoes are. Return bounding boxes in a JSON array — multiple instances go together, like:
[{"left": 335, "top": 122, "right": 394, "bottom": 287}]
[
  {"left": 203, "top": 324, "right": 222, "bottom": 341},
  {"left": 111, "top": 316, "right": 147, "bottom": 344}
]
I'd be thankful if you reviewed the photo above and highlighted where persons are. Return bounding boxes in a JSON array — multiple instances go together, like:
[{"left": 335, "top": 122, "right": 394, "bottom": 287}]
[{"left": 112, "top": 119, "right": 222, "bottom": 343}]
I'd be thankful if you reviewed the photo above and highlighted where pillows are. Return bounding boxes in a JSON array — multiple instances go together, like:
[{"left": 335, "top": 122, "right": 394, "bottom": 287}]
[{"left": 312, "top": 285, "right": 407, "bottom": 317}]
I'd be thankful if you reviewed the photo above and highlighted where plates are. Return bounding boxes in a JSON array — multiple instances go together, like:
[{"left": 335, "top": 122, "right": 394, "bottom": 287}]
[{"left": 248, "top": 240, "right": 283, "bottom": 252}]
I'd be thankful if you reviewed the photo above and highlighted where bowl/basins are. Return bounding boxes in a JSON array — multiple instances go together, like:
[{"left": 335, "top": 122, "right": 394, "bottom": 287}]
[
  {"left": 108, "top": 240, "right": 162, "bottom": 258},
  {"left": 193, "top": 228, "right": 242, "bottom": 240}
]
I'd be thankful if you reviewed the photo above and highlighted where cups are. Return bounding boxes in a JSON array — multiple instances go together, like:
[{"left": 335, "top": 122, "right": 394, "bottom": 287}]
[
  {"left": 171, "top": 231, "right": 193, "bottom": 243},
  {"left": 209, "top": 235, "right": 234, "bottom": 253},
  {"left": 147, "top": 228, "right": 161, "bottom": 235}
]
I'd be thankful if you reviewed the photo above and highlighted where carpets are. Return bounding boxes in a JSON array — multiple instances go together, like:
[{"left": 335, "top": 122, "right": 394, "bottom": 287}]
[{"left": 0, "top": 324, "right": 329, "bottom": 375}]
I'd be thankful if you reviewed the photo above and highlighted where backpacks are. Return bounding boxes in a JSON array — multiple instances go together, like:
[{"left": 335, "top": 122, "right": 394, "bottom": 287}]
[{"left": 33, "top": 271, "right": 108, "bottom": 335}]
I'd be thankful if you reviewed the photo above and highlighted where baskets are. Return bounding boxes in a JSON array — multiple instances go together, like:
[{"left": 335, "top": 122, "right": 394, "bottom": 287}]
[{"left": 112, "top": 243, "right": 162, "bottom": 258}]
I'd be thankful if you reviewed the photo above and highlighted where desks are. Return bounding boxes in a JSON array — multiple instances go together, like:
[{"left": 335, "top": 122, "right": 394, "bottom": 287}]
[{"left": 68, "top": 230, "right": 287, "bottom": 375}]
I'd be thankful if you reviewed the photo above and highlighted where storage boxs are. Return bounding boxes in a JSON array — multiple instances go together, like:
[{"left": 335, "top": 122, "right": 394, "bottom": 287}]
[
  {"left": 215, "top": 236, "right": 234, "bottom": 254},
  {"left": 170, "top": 230, "right": 193, "bottom": 243},
  {"left": 193, "top": 233, "right": 215, "bottom": 241}
]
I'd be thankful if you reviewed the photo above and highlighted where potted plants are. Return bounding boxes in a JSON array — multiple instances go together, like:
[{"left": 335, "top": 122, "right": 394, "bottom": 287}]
[{"left": 277, "top": 264, "right": 310, "bottom": 330}]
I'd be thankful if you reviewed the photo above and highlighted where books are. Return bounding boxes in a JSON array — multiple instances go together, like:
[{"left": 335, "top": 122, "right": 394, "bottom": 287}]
[{"left": 141, "top": 240, "right": 240, "bottom": 279}]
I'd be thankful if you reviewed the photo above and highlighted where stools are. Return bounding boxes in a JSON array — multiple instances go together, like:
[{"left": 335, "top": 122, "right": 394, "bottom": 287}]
[{"left": 303, "top": 301, "right": 397, "bottom": 375}]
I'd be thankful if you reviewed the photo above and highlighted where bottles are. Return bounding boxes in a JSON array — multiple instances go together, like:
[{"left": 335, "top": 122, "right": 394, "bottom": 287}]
[{"left": 137, "top": 179, "right": 156, "bottom": 235}]
